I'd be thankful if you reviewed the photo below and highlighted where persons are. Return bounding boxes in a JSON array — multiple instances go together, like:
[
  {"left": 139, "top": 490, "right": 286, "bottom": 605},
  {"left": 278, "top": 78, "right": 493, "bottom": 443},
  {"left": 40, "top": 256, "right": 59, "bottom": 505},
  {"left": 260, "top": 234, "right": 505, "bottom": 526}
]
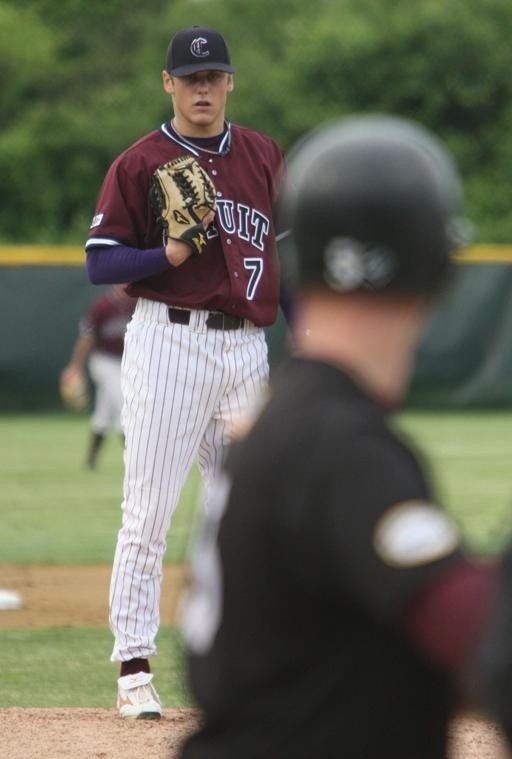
[
  {"left": 60, "top": 282, "right": 139, "bottom": 464},
  {"left": 85, "top": 26, "right": 295, "bottom": 716},
  {"left": 173, "top": 109, "right": 510, "bottom": 757}
]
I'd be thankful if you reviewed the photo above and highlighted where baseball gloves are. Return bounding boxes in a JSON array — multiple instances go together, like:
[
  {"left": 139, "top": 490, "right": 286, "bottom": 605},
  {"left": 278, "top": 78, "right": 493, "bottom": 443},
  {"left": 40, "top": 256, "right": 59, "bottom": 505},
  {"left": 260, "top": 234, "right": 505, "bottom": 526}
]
[
  {"left": 61, "top": 369, "right": 90, "bottom": 408},
  {"left": 148, "top": 155, "right": 216, "bottom": 257}
]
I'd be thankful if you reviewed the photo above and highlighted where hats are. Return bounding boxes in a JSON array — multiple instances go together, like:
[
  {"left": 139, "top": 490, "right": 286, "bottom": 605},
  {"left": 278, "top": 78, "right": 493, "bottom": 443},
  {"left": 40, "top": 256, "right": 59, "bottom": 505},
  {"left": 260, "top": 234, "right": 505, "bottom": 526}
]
[{"left": 164, "top": 21, "right": 236, "bottom": 78}]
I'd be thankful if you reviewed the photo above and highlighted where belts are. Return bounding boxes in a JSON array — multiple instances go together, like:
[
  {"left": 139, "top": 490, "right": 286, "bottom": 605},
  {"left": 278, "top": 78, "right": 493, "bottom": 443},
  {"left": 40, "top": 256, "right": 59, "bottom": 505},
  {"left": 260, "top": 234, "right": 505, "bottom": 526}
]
[{"left": 167, "top": 305, "right": 245, "bottom": 334}]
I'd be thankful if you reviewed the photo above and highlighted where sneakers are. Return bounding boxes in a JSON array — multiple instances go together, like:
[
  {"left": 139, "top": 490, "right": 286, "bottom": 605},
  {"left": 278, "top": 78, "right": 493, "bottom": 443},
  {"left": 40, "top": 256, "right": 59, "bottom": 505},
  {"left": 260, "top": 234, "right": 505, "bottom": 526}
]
[
  {"left": 1, "top": 588, "right": 22, "bottom": 610},
  {"left": 116, "top": 675, "right": 164, "bottom": 721}
]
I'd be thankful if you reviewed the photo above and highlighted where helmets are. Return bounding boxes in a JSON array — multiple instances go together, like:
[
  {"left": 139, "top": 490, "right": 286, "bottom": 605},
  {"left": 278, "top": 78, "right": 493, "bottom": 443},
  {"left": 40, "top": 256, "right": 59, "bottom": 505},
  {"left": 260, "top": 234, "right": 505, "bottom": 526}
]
[{"left": 274, "top": 116, "right": 467, "bottom": 308}]
